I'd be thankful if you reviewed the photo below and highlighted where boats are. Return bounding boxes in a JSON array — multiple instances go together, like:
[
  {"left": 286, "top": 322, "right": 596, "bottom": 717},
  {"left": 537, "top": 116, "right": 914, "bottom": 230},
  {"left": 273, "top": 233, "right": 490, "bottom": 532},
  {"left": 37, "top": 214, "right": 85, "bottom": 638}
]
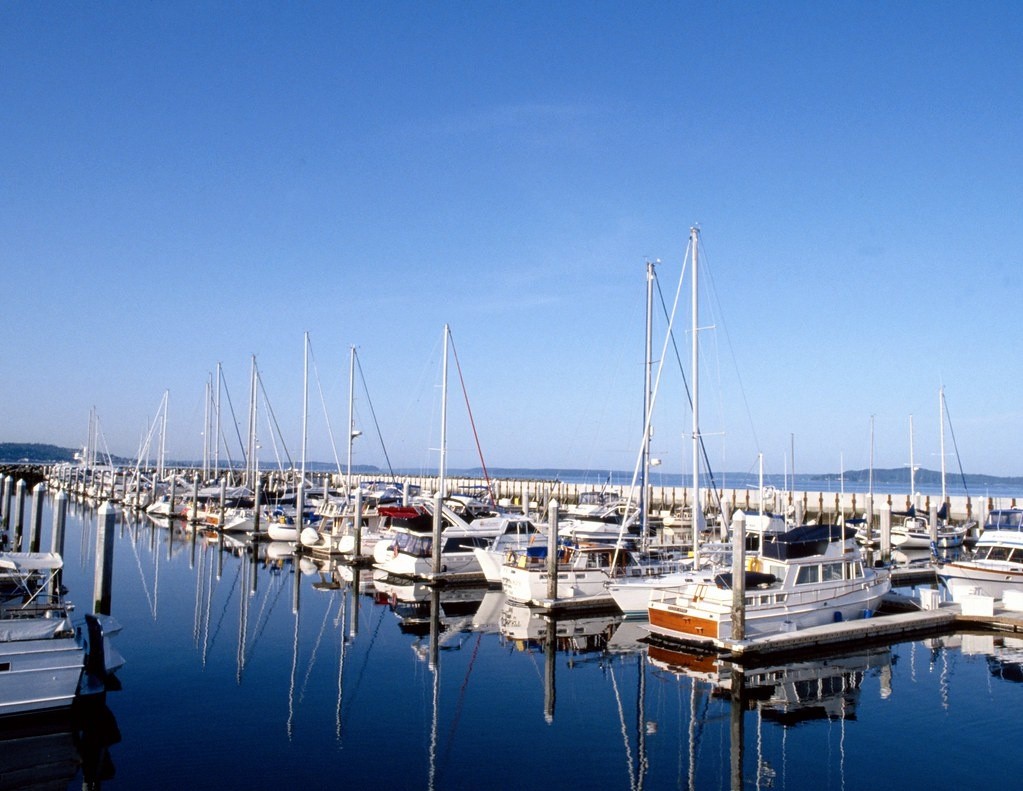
[
  {"left": 662, "top": 503, "right": 716, "bottom": 526},
  {"left": 938, "top": 506, "right": 1023, "bottom": 617},
  {"left": 564, "top": 491, "right": 625, "bottom": 521},
  {"left": 0, "top": 552, "right": 86, "bottom": 718},
  {"left": 733, "top": 452, "right": 787, "bottom": 536}
]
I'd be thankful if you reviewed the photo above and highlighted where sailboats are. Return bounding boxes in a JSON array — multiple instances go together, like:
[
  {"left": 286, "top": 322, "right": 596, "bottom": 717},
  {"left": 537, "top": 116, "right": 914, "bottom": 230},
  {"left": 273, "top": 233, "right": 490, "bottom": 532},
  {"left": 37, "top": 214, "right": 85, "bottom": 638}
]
[
  {"left": 45, "top": 226, "right": 977, "bottom": 661},
  {"left": 49, "top": 489, "right": 901, "bottom": 791}
]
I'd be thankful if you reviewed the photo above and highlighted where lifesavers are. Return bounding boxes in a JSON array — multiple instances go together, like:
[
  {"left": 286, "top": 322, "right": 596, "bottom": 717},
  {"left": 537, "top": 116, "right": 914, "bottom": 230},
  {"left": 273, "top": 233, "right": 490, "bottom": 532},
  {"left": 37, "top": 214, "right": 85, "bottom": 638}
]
[
  {"left": 747, "top": 557, "right": 760, "bottom": 573},
  {"left": 507, "top": 551, "right": 518, "bottom": 563}
]
